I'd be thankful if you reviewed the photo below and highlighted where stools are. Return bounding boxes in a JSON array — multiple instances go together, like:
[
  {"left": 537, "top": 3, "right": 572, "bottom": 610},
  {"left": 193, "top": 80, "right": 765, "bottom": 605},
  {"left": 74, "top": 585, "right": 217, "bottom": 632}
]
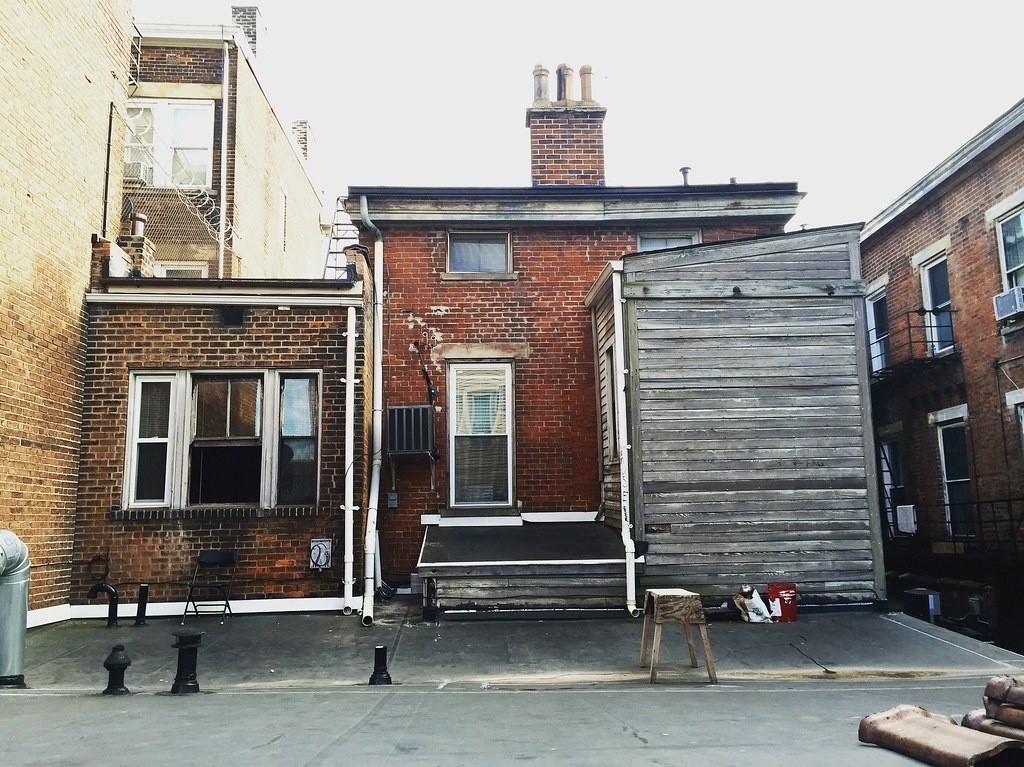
[{"left": 640, "top": 586, "right": 718, "bottom": 683}]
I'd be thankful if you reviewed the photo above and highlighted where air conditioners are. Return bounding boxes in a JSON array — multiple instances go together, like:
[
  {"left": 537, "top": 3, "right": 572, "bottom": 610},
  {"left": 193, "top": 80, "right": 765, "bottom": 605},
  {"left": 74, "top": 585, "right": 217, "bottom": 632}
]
[{"left": 991, "top": 288, "right": 1024, "bottom": 322}]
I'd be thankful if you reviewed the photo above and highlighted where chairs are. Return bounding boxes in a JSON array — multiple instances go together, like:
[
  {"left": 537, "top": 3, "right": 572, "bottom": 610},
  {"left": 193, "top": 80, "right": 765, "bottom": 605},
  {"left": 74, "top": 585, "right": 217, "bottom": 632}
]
[{"left": 180, "top": 548, "right": 239, "bottom": 626}]
